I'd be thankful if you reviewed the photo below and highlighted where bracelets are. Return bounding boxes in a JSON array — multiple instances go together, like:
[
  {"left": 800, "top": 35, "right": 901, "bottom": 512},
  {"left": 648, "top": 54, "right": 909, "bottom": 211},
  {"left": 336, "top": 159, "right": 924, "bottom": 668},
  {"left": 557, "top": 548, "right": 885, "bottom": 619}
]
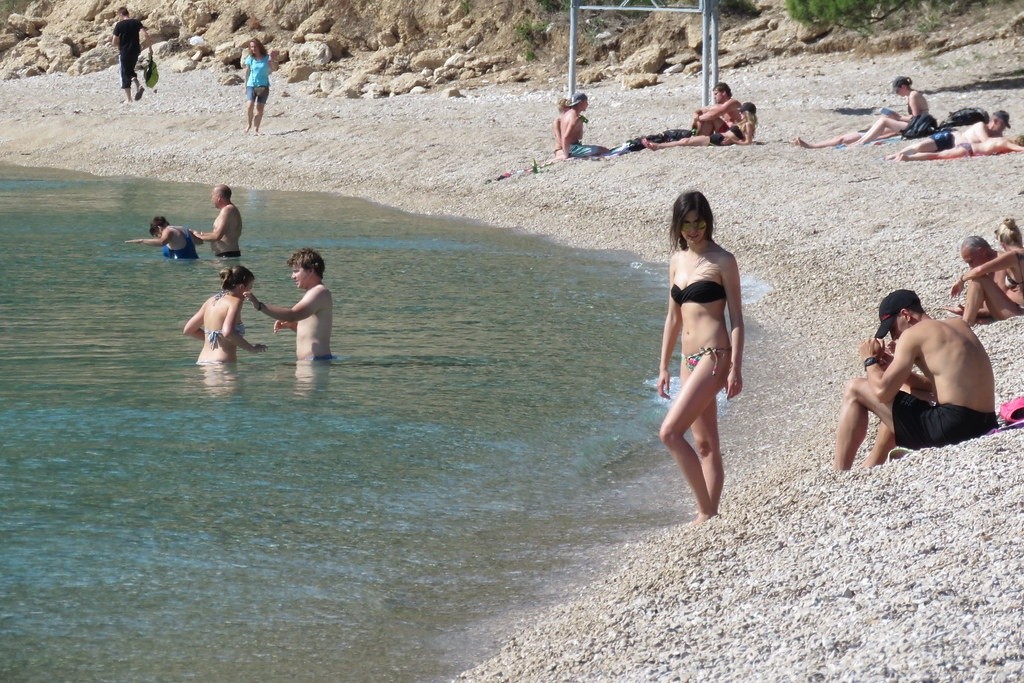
[{"left": 960, "top": 274, "right": 965, "bottom": 282}]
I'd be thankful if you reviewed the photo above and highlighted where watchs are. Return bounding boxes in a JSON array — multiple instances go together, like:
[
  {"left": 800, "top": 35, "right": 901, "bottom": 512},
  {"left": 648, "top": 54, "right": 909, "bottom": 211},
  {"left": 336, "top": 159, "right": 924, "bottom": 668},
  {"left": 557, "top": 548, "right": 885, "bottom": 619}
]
[
  {"left": 257, "top": 302, "right": 265, "bottom": 311},
  {"left": 864, "top": 356, "right": 880, "bottom": 371}
]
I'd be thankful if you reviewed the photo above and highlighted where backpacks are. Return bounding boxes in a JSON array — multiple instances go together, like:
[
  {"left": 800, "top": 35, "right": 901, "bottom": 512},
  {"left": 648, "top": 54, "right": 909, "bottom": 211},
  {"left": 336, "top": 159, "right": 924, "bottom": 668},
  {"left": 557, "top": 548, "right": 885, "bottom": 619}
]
[
  {"left": 943, "top": 106, "right": 990, "bottom": 128},
  {"left": 901, "top": 114, "right": 938, "bottom": 139},
  {"left": 143, "top": 52, "right": 159, "bottom": 88}
]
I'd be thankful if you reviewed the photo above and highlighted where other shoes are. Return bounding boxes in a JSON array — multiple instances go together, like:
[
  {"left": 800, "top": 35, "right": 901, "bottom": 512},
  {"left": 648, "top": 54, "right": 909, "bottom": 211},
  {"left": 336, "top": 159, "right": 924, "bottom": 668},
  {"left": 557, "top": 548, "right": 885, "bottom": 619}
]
[
  {"left": 641, "top": 137, "right": 653, "bottom": 151},
  {"left": 134, "top": 86, "right": 144, "bottom": 101}
]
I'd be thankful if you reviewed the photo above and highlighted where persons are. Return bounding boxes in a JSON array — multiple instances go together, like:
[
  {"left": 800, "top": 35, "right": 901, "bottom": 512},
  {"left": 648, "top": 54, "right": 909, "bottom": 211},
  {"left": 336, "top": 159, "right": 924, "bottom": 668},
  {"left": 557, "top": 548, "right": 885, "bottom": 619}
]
[
  {"left": 189, "top": 181, "right": 242, "bottom": 258},
  {"left": 182, "top": 265, "right": 268, "bottom": 363},
  {"left": 788, "top": 124, "right": 896, "bottom": 148},
  {"left": 241, "top": 247, "right": 333, "bottom": 361},
  {"left": 941, "top": 217, "right": 1024, "bottom": 327},
  {"left": 112, "top": 7, "right": 153, "bottom": 104},
  {"left": 834, "top": 290, "right": 1000, "bottom": 470},
  {"left": 244, "top": 38, "right": 279, "bottom": 135},
  {"left": 842, "top": 74, "right": 928, "bottom": 147},
  {"left": 124, "top": 215, "right": 204, "bottom": 260},
  {"left": 884, "top": 110, "right": 1024, "bottom": 163},
  {"left": 693, "top": 82, "right": 742, "bottom": 137},
  {"left": 552, "top": 92, "right": 609, "bottom": 159},
  {"left": 641, "top": 101, "right": 757, "bottom": 149},
  {"left": 657, "top": 192, "right": 745, "bottom": 526}
]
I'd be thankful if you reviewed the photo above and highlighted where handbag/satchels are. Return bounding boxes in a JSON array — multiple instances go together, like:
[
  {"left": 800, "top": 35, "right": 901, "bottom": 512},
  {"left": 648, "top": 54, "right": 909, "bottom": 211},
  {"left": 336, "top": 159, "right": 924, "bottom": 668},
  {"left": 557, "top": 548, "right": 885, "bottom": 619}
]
[{"left": 253, "top": 86, "right": 266, "bottom": 96}]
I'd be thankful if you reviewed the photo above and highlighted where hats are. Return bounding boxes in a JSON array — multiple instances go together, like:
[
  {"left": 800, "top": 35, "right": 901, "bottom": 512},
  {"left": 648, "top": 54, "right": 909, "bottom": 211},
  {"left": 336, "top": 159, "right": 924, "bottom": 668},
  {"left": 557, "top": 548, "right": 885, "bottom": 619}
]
[
  {"left": 737, "top": 102, "right": 756, "bottom": 114},
  {"left": 569, "top": 92, "right": 588, "bottom": 107},
  {"left": 993, "top": 109, "right": 1011, "bottom": 128},
  {"left": 874, "top": 289, "right": 919, "bottom": 339}
]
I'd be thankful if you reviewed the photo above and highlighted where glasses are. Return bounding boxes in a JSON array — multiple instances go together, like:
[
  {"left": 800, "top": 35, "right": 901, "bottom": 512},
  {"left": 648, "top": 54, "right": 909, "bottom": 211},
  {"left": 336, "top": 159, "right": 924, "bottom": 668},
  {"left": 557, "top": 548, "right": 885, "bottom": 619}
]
[{"left": 679, "top": 218, "right": 706, "bottom": 232}]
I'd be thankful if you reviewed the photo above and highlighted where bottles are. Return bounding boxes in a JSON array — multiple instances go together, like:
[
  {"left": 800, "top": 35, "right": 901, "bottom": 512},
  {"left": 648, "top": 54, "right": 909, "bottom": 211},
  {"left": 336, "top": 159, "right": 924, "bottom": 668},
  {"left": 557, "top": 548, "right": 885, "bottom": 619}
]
[
  {"left": 532, "top": 159, "right": 538, "bottom": 173},
  {"left": 692, "top": 127, "right": 697, "bottom": 134},
  {"left": 272, "top": 47, "right": 280, "bottom": 62},
  {"left": 578, "top": 113, "right": 588, "bottom": 123}
]
